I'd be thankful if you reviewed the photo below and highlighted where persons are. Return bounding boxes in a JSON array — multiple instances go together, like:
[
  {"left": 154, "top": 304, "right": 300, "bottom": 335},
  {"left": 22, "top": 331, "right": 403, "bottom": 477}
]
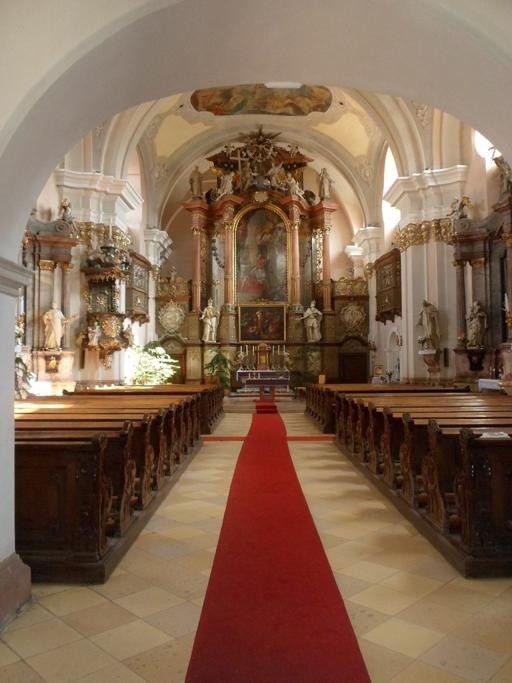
[
  {"left": 465, "top": 301, "right": 487, "bottom": 346},
  {"left": 302, "top": 300, "right": 320, "bottom": 341},
  {"left": 417, "top": 299, "right": 438, "bottom": 337},
  {"left": 197, "top": 298, "right": 218, "bottom": 343},
  {"left": 42, "top": 302, "right": 64, "bottom": 350}
]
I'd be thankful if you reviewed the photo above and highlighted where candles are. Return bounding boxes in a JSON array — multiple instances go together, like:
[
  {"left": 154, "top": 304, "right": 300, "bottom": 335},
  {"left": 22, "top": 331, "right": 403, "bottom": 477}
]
[
  {"left": 252, "top": 346, "right": 255, "bottom": 356},
  {"left": 246, "top": 345, "right": 248, "bottom": 355},
  {"left": 273, "top": 345, "right": 274, "bottom": 355},
  {"left": 240, "top": 346, "right": 242, "bottom": 353},
  {"left": 283, "top": 345, "right": 285, "bottom": 353},
  {"left": 277, "top": 345, "right": 280, "bottom": 353}
]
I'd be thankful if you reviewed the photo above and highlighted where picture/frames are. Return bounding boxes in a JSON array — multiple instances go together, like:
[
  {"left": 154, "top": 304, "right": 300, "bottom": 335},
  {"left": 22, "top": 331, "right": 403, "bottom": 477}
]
[{"left": 237, "top": 303, "right": 287, "bottom": 344}]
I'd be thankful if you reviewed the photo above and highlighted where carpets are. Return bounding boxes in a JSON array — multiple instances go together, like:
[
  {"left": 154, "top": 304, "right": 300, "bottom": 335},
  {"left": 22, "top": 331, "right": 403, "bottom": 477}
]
[{"left": 184, "top": 400, "right": 371, "bottom": 682}]
